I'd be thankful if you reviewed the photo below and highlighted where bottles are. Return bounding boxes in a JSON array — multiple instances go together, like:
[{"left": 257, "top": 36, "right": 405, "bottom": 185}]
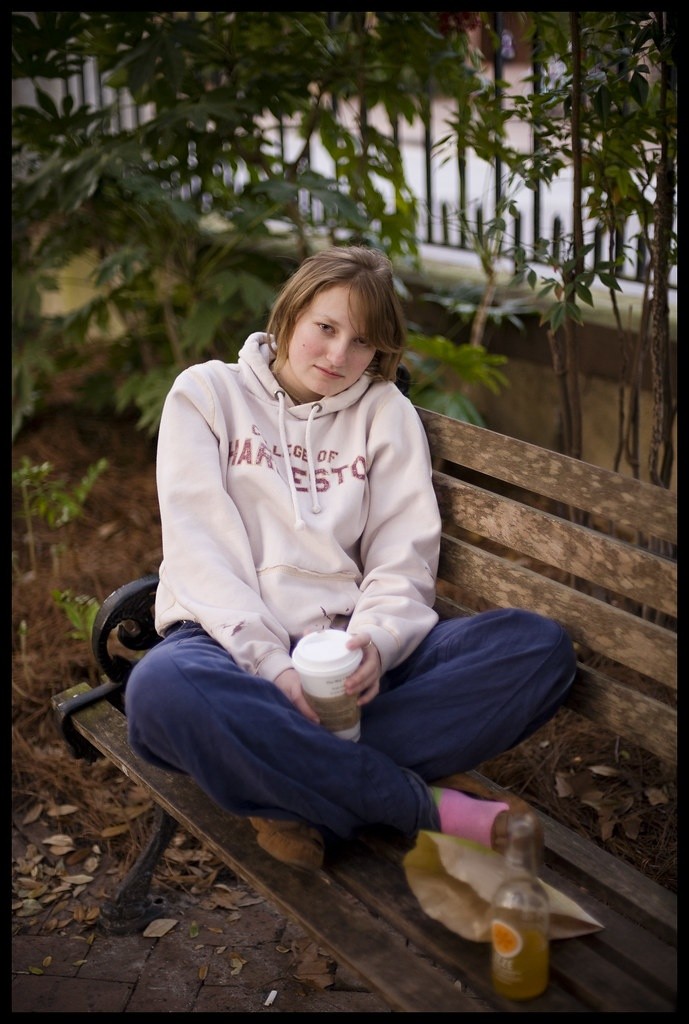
[{"left": 492, "top": 823, "right": 548, "bottom": 1001}]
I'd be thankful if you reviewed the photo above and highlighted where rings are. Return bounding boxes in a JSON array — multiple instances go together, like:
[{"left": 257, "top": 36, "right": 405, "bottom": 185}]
[{"left": 363, "top": 640, "right": 371, "bottom": 648}]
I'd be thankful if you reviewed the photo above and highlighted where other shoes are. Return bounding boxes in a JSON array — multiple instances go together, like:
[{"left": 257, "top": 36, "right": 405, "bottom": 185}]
[
  {"left": 251, "top": 816, "right": 325, "bottom": 868},
  {"left": 491, "top": 796, "right": 545, "bottom": 870}
]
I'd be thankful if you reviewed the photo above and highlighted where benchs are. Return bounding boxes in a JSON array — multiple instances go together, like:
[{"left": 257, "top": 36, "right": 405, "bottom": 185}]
[{"left": 52, "top": 405, "right": 676, "bottom": 1013}]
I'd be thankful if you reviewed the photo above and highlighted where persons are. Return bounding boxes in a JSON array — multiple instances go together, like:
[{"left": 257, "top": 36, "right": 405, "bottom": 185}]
[{"left": 126, "top": 246, "right": 577, "bottom": 871}]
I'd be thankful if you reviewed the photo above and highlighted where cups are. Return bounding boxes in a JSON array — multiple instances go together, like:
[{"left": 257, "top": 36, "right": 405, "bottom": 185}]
[{"left": 292, "top": 629, "right": 363, "bottom": 743}]
